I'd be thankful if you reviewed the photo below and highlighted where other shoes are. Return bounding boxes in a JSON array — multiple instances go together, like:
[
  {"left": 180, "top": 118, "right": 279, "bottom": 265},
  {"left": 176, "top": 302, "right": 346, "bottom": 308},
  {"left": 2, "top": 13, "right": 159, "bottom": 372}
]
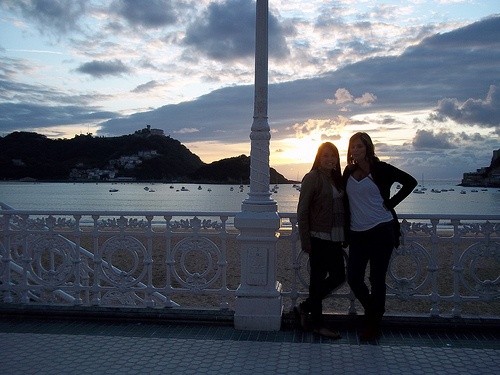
[
  {"left": 293, "top": 303, "right": 311, "bottom": 332},
  {"left": 357, "top": 306, "right": 385, "bottom": 341},
  {"left": 312, "top": 325, "right": 341, "bottom": 339}
]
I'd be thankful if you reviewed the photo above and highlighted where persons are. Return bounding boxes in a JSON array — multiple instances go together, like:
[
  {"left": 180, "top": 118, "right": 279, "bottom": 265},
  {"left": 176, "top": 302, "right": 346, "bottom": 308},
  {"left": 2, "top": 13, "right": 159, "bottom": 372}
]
[
  {"left": 340, "top": 132, "right": 419, "bottom": 345},
  {"left": 281, "top": 142, "right": 348, "bottom": 339}
]
